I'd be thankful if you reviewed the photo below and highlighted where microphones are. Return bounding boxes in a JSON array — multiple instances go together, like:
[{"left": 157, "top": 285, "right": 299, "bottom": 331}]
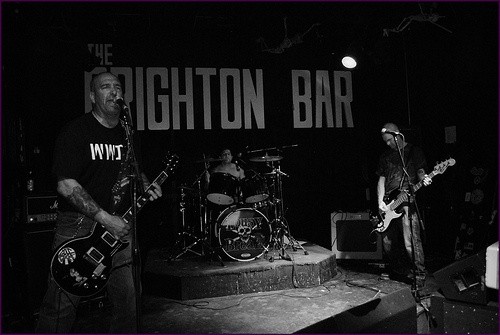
[
  {"left": 383, "top": 129, "right": 402, "bottom": 135},
  {"left": 114, "top": 95, "right": 128, "bottom": 109}
]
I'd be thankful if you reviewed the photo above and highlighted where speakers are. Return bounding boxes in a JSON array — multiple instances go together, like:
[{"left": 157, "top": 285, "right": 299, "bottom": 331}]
[
  {"left": 288, "top": 287, "right": 417, "bottom": 334},
  {"left": 434, "top": 240, "right": 500, "bottom": 303}
]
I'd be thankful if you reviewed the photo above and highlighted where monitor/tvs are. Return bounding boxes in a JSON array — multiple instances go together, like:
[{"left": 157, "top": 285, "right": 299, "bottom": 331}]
[{"left": 330, "top": 213, "right": 383, "bottom": 260}]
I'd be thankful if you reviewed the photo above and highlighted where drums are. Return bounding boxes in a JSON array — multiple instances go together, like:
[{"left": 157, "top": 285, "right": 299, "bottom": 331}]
[
  {"left": 207, "top": 172, "right": 238, "bottom": 205},
  {"left": 215, "top": 205, "right": 273, "bottom": 261},
  {"left": 239, "top": 177, "right": 270, "bottom": 204}
]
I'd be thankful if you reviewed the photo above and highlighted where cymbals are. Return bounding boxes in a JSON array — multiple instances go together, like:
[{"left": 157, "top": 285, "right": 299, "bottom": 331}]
[
  {"left": 194, "top": 157, "right": 227, "bottom": 162},
  {"left": 249, "top": 156, "right": 282, "bottom": 162}
]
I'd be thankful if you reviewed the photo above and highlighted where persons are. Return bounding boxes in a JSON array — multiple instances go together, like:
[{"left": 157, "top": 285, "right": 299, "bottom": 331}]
[
  {"left": 205, "top": 149, "right": 245, "bottom": 190},
  {"left": 377, "top": 123, "right": 432, "bottom": 287},
  {"left": 36, "top": 73, "right": 163, "bottom": 334}
]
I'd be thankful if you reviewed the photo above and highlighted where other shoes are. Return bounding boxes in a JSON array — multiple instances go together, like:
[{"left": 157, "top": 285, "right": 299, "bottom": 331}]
[{"left": 410, "top": 278, "right": 426, "bottom": 290}]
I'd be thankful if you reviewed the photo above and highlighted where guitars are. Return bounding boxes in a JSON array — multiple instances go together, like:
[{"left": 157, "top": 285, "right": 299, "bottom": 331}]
[
  {"left": 370, "top": 157, "right": 456, "bottom": 233},
  {"left": 50, "top": 152, "right": 179, "bottom": 298}
]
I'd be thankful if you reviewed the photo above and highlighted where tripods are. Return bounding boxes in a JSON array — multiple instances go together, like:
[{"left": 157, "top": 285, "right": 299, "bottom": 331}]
[
  {"left": 247, "top": 145, "right": 308, "bottom": 263},
  {"left": 167, "top": 162, "right": 228, "bottom": 267}
]
